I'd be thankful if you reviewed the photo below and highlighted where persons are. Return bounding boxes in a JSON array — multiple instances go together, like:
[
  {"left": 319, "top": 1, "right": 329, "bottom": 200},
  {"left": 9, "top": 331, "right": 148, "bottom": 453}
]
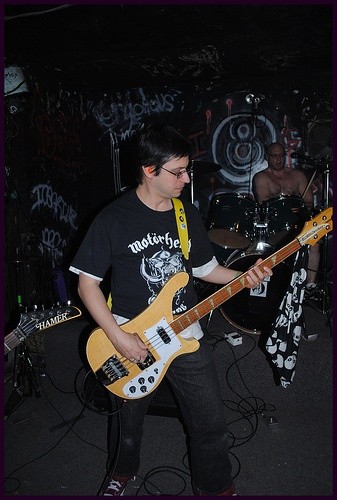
[
  {"left": 68, "top": 125, "right": 273, "bottom": 497},
  {"left": 252, "top": 143, "right": 328, "bottom": 301}
]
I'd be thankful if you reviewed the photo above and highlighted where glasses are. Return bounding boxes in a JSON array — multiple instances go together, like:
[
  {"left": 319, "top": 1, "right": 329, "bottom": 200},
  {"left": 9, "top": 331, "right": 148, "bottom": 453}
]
[
  {"left": 267, "top": 153, "right": 284, "bottom": 158},
  {"left": 160, "top": 167, "right": 189, "bottom": 179}
]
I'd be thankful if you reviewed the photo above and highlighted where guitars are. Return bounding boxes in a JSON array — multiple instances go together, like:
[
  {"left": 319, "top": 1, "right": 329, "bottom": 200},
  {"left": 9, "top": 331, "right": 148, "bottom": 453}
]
[
  {"left": 85, "top": 205, "right": 337, "bottom": 399},
  {"left": 4, "top": 299, "right": 83, "bottom": 353}
]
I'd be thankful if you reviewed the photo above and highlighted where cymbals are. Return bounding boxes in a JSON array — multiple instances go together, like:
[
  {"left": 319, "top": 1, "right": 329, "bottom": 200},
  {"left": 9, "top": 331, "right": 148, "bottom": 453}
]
[{"left": 187, "top": 160, "right": 222, "bottom": 179}]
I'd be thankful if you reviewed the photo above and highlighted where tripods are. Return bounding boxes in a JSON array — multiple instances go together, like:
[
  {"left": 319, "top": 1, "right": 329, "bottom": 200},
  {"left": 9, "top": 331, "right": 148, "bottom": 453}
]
[{"left": 303, "top": 162, "right": 332, "bottom": 314}]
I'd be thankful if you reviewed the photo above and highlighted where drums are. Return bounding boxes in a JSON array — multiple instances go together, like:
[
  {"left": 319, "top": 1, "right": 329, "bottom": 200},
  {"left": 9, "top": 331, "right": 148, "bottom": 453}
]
[
  {"left": 261, "top": 192, "right": 311, "bottom": 247},
  {"left": 205, "top": 192, "right": 258, "bottom": 248},
  {"left": 219, "top": 253, "right": 294, "bottom": 335}
]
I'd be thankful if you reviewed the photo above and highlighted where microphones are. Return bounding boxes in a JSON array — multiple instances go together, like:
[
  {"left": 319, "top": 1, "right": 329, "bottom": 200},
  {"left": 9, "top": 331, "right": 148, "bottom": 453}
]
[
  {"left": 245, "top": 93, "right": 261, "bottom": 104},
  {"left": 291, "top": 151, "right": 316, "bottom": 161}
]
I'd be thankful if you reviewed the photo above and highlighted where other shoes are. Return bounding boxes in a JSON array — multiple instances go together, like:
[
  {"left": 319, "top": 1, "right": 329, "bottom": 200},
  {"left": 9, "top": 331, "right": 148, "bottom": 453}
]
[{"left": 101, "top": 476, "right": 132, "bottom": 498}]
